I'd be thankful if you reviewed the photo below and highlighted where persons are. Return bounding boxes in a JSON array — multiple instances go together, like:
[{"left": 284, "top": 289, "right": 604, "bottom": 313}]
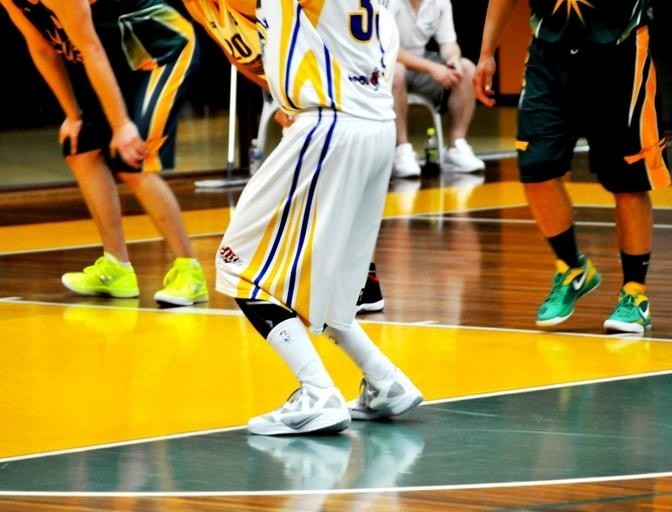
[
  {"left": 214, "top": 0, "right": 422, "bottom": 436},
  {"left": 385, "top": 0, "right": 486, "bottom": 178},
  {"left": 0, "top": 0, "right": 209, "bottom": 306},
  {"left": 183, "top": 0, "right": 386, "bottom": 312},
  {"left": 472, "top": 0, "right": 672, "bottom": 333}
]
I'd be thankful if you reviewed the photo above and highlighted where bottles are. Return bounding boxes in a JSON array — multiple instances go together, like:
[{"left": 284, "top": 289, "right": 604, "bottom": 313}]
[
  {"left": 248, "top": 138, "right": 264, "bottom": 178},
  {"left": 423, "top": 127, "right": 442, "bottom": 178}
]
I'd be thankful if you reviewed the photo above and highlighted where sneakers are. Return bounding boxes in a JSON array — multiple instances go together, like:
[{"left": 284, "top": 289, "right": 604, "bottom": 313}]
[
  {"left": 247, "top": 436, "right": 424, "bottom": 477},
  {"left": 445, "top": 139, "right": 485, "bottom": 173},
  {"left": 604, "top": 281, "right": 652, "bottom": 332},
  {"left": 61, "top": 256, "right": 139, "bottom": 298},
  {"left": 536, "top": 255, "right": 601, "bottom": 326},
  {"left": 154, "top": 258, "right": 208, "bottom": 306},
  {"left": 392, "top": 143, "right": 422, "bottom": 177},
  {"left": 247, "top": 369, "right": 423, "bottom": 434},
  {"left": 356, "top": 262, "right": 384, "bottom": 315}
]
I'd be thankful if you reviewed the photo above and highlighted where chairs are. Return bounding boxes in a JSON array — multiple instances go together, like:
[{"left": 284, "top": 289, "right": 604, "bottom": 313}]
[{"left": 256, "top": 85, "right": 444, "bottom": 161}]
